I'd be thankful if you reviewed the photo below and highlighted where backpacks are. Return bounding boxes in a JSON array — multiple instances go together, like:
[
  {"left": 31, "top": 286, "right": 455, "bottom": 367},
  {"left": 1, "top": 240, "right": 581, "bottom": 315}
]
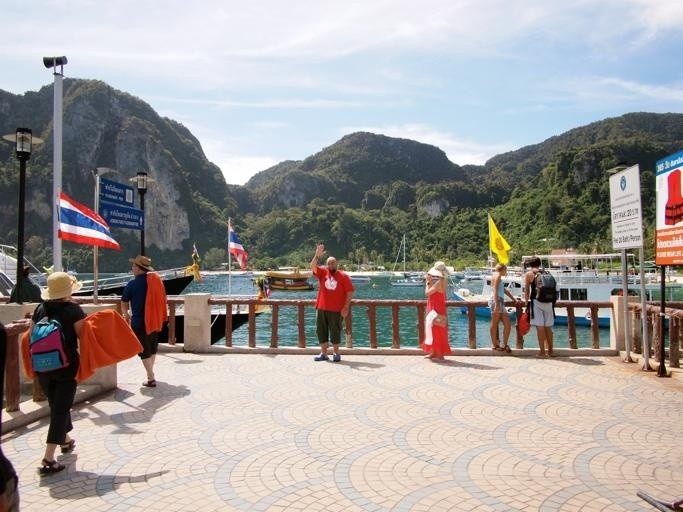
[
  {"left": 532, "top": 270, "right": 556, "bottom": 304},
  {"left": 28, "top": 301, "right": 80, "bottom": 373}
]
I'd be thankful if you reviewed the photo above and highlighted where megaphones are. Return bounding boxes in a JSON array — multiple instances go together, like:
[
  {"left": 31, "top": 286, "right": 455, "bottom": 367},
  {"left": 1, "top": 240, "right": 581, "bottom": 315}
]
[{"left": 43, "top": 56, "right": 67, "bottom": 68}]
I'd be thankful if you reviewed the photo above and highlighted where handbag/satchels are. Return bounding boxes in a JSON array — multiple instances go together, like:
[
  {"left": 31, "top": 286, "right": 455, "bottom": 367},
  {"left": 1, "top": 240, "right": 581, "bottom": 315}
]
[
  {"left": 517, "top": 311, "right": 531, "bottom": 336},
  {"left": 432, "top": 313, "right": 448, "bottom": 329}
]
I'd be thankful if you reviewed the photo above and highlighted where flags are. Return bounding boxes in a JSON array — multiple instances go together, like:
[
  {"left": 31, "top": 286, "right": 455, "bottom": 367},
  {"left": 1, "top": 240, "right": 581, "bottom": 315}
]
[
  {"left": 488, "top": 216, "right": 512, "bottom": 265},
  {"left": 228, "top": 222, "right": 248, "bottom": 270},
  {"left": 57, "top": 192, "right": 122, "bottom": 253}
]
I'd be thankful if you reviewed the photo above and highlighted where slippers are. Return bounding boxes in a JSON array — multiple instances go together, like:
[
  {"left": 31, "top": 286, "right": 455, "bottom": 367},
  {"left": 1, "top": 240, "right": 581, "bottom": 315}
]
[
  {"left": 313, "top": 352, "right": 329, "bottom": 362},
  {"left": 491, "top": 345, "right": 560, "bottom": 359},
  {"left": 332, "top": 353, "right": 341, "bottom": 362}
]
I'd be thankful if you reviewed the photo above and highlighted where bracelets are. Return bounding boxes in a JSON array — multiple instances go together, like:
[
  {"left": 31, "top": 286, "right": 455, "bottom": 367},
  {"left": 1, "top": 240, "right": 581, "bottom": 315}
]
[
  {"left": 426, "top": 282, "right": 431, "bottom": 285},
  {"left": 122, "top": 313, "right": 129, "bottom": 318},
  {"left": 313, "top": 255, "right": 320, "bottom": 261}
]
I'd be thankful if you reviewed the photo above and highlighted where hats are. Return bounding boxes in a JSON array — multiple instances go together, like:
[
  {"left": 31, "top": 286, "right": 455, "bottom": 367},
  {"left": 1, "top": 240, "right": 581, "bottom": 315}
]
[
  {"left": 39, "top": 272, "right": 82, "bottom": 301},
  {"left": 128, "top": 252, "right": 157, "bottom": 272},
  {"left": 428, "top": 261, "right": 453, "bottom": 279}
]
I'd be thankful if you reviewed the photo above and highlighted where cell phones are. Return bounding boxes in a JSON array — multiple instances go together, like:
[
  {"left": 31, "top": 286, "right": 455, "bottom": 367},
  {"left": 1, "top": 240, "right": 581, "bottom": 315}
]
[{"left": 427, "top": 272, "right": 430, "bottom": 280}]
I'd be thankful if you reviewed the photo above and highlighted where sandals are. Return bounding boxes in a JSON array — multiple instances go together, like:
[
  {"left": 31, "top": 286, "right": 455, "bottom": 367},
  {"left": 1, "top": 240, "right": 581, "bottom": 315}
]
[
  {"left": 61, "top": 433, "right": 76, "bottom": 454},
  {"left": 142, "top": 379, "right": 157, "bottom": 387},
  {"left": 39, "top": 458, "right": 65, "bottom": 474},
  {"left": 424, "top": 354, "right": 445, "bottom": 362}
]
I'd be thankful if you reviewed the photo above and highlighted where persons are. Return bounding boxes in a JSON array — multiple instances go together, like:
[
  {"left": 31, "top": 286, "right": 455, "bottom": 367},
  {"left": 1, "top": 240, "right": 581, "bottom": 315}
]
[
  {"left": 489, "top": 263, "right": 516, "bottom": 353},
  {"left": 563, "top": 267, "right": 572, "bottom": 272},
  {"left": 307, "top": 243, "right": 353, "bottom": 361},
  {"left": 524, "top": 257, "right": 554, "bottom": 358},
  {"left": 508, "top": 280, "right": 516, "bottom": 288},
  {"left": 575, "top": 260, "right": 583, "bottom": 271},
  {"left": 422, "top": 259, "right": 452, "bottom": 360},
  {"left": 121, "top": 255, "right": 168, "bottom": 386},
  {"left": 6, "top": 266, "right": 43, "bottom": 303},
  {"left": 0, "top": 325, "right": 23, "bottom": 511},
  {"left": 27, "top": 271, "right": 88, "bottom": 476}
]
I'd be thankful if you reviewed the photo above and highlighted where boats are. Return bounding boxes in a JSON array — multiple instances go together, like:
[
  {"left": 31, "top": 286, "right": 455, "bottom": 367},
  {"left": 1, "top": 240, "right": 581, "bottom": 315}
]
[
  {"left": 0, "top": 240, "right": 274, "bottom": 347},
  {"left": 343, "top": 274, "right": 372, "bottom": 288},
  {"left": 252, "top": 257, "right": 314, "bottom": 295},
  {"left": 387, "top": 231, "right": 683, "bottom": 335}
]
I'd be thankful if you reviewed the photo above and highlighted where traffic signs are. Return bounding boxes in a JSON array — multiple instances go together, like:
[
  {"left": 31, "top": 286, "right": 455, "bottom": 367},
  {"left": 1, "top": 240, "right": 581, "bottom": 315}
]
[
  {"left": 99, "top": 177, "right": 135, "bottom": 209},
  {"left": 97, "top": 206, "right": 145, "bottom": 230}
]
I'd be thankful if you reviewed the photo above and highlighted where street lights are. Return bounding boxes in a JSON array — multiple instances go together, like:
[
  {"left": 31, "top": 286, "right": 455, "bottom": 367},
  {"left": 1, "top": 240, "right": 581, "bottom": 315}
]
[
  {"left": 2, "top": 126, "right": 43, "bottom": 303},
  {"left": 131, "top": 164, "right": 150, "bottom": 279},
  {"left": 39, "top": 56, "right": 69, "bottom": 287},
  {"left": 87, "top": 165, "right": 121, "bottom": 305}
]
